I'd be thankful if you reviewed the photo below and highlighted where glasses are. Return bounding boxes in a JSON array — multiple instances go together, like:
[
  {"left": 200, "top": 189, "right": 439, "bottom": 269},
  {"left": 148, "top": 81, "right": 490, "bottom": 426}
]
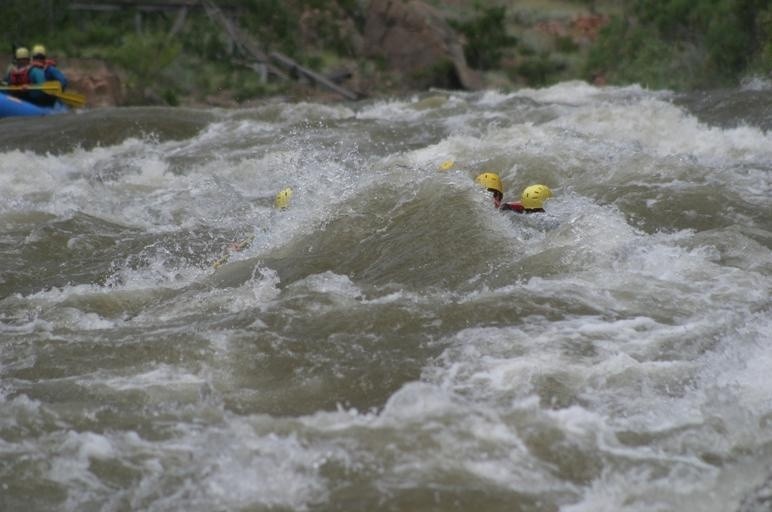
[
  {"left": 36, "top": 55, "right": 45, "bottom": 59},
  {"left": 491, "top": 190, "right": 503, "bottom": 199}
]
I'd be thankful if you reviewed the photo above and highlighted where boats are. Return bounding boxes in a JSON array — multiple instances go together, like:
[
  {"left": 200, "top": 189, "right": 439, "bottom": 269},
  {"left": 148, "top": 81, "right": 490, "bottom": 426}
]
[{"left": 1, "top": 94, "right": 70, "bottom": 117}]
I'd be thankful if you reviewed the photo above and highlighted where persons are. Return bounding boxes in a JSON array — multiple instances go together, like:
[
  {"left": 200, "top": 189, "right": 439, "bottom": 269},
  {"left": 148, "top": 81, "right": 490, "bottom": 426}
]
[
  {"left": 475, "top": 172, "right": 553, "bottom": 215},
  {"left": 2, "top": 44, "right": 68, "bottom": 91}
]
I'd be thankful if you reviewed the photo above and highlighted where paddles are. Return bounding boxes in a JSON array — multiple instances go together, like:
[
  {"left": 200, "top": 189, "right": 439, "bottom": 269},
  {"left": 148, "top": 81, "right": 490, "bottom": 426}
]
[
  {"left": 56, "top": 92, "right": 85, "bottom": 110},
  {"left": 0, "top": 80, "right": 61, "bottom": 95}
]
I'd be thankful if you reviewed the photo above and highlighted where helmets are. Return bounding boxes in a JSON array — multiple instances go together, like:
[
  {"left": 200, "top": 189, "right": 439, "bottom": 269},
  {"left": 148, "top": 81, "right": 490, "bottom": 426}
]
[
  {"left": 473, "top": 172, "right": 502, "bottom": 194},
  {"left": 440, "top": 161, "right": 456, "bottom": 171},
  {"left": 272, "top": 188, "right": 292, "bottom": 210},
  {"left": 32, "top": 45, "right": 48, "bottom": 57},
  {"left": 16, "top": 47, "right": 29, "bottom": 59},
  {"left": 521, "top": 185, "right": 553, "bottom": 211}
]
